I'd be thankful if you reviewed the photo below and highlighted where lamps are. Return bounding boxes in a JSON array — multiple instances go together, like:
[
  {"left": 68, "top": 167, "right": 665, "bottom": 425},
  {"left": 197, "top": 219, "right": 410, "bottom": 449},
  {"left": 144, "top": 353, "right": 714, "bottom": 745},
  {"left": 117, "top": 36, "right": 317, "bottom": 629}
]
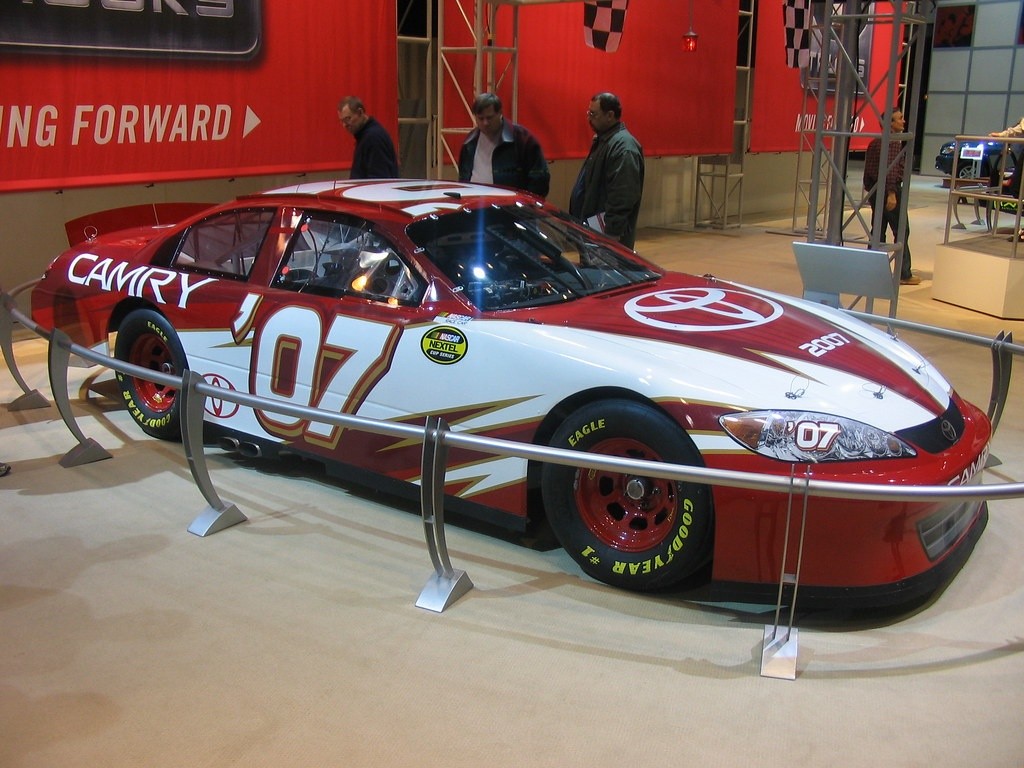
[{"left": 681, "top": 0, "right": 699, "bottom": 54}]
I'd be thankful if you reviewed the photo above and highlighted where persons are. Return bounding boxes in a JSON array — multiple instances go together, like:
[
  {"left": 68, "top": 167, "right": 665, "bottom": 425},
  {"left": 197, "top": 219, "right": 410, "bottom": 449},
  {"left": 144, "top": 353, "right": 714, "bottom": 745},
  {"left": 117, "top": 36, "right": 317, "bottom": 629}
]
[
  {"left": 989, "top": 118, "right": 1024, "bottom": 242},
  {"left": 337, "top": 95, "right": 399, "bottom": 179},
  {"left": 863, "top": 107, "right": 922, "bottom": 284},
  {"left": 458, "top": 92, "right": 551, "bottom": 198},
  {"left": 569, "top": 93, "right": 645, "bottom": 251}
]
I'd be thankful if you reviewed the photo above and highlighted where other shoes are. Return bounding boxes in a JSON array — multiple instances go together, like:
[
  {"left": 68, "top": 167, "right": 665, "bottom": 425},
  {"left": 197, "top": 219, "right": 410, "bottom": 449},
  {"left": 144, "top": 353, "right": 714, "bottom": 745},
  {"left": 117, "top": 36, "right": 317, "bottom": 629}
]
[{"left": 1007, "top": 227, "right": 1024, "bottom": 243}]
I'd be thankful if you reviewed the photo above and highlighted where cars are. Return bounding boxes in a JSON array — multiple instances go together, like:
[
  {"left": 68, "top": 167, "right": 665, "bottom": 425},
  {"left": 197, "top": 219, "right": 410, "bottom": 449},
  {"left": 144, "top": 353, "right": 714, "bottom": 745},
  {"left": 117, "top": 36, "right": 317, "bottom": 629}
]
[
  {"left": 932, "top": 133, "right": 1024, "bottom": 214},
  {"left": 30, "top": 177, "right": 1003, "bottom": 611}
]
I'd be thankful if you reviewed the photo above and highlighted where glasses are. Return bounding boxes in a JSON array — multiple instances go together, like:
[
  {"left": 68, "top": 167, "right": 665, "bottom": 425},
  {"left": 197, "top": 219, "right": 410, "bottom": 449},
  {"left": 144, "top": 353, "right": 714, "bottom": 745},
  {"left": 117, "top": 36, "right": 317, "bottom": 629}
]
[{"left": 587, "top": 110, "right": 604, "bottom": 119}]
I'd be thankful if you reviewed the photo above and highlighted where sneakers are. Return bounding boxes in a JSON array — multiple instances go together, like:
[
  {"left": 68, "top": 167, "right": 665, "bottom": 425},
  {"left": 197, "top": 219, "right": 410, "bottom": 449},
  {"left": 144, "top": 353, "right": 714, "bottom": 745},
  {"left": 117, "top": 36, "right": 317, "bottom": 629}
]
[{"left": 899, "top": 274, "right": 922, "bottom": 285}]
